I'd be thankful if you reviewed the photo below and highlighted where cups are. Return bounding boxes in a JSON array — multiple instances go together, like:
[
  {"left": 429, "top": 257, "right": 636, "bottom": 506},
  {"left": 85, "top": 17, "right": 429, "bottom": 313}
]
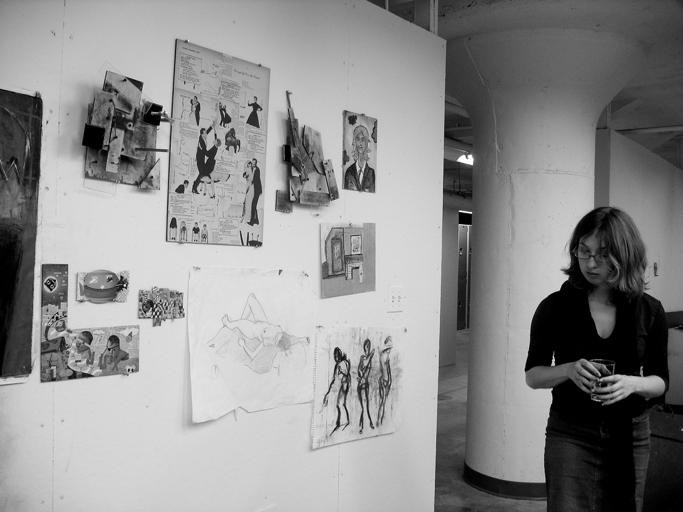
[{"left": 588, "top": 357, "right": 616, "bottom": 403}]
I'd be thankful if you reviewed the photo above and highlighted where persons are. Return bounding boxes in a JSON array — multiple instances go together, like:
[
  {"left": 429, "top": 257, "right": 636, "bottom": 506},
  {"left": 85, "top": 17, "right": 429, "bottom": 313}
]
[
  {"left": 323, "top": 334, "right": 394, "bottom": 433},
  {"left": 525, "top": 207, "right": 669, "bottom": 512},
  {"left": 247, "top": 95, "right": 263, "bottom": 129},
  {"left": 169, "top": 217, "right": 209, "bottom": 244},
  {"left": 175, "top": 95, "right": 240, "bottom": 196},
  {"left": 242, "top": 159, "right": 262, "bottom": 225},
  {"left": 344, "top": 126, "right": 374, "bottom": 192},
  {"left": 70, "top": 332, "right": 129, "bottom": 372}
]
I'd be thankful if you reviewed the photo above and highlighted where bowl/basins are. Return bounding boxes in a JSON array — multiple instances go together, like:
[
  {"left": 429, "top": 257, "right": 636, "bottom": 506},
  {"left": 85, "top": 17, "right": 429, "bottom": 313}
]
[{"left": 86, "top": 269, "right": 118, "bottom": 303}]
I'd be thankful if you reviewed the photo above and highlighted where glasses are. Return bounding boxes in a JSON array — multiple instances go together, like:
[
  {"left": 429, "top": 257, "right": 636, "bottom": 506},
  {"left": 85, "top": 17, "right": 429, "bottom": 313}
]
[{"left": 572, "top": 246, "right": 611, "bottom": 265}]
